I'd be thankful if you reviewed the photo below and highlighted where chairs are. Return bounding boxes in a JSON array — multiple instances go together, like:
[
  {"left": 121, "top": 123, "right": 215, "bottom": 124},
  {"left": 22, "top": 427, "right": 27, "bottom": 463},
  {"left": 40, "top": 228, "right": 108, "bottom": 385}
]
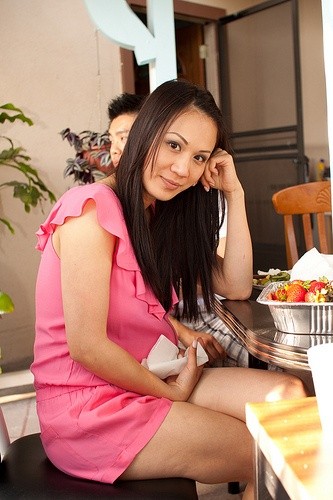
[
  {"left": 271, "top": 181, "right": 333, "bottom": 271},
  {"left": 0, "top": 405, "right": 201, "bottom": 500}
]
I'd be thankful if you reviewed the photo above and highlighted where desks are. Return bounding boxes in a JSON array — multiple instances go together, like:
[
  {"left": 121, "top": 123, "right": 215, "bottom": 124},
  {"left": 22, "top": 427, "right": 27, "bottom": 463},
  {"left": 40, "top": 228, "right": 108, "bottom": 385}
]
[
  {"left": 244, "top": 397, "right": 333, "bottom": 500},
  {"left": 208, "top": 275, "right": 333, "bottom": 495}
]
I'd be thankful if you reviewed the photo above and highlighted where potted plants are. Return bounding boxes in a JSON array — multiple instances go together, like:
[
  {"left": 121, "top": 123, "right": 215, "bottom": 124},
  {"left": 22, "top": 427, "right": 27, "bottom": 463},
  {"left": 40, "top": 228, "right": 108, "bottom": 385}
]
[{"left": 58, "top": 127, "right": 116, "bottom": 185}]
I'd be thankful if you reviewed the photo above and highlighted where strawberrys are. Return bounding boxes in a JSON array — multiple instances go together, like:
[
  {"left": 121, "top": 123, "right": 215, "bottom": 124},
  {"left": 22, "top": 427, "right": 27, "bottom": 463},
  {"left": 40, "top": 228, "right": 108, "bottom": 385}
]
[{"left": 266, "top": 274, "right": 332, "bottom": 305}]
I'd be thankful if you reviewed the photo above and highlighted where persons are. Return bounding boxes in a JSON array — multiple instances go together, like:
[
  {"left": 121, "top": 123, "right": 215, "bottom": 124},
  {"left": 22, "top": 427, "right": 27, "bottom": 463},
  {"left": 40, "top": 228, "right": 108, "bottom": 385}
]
[
  {"left": 30, "top": 75, "right": 307, "bottom": 500},
  {"left": 107, "top": 92, "right": 250, "bottom": 368}
]
